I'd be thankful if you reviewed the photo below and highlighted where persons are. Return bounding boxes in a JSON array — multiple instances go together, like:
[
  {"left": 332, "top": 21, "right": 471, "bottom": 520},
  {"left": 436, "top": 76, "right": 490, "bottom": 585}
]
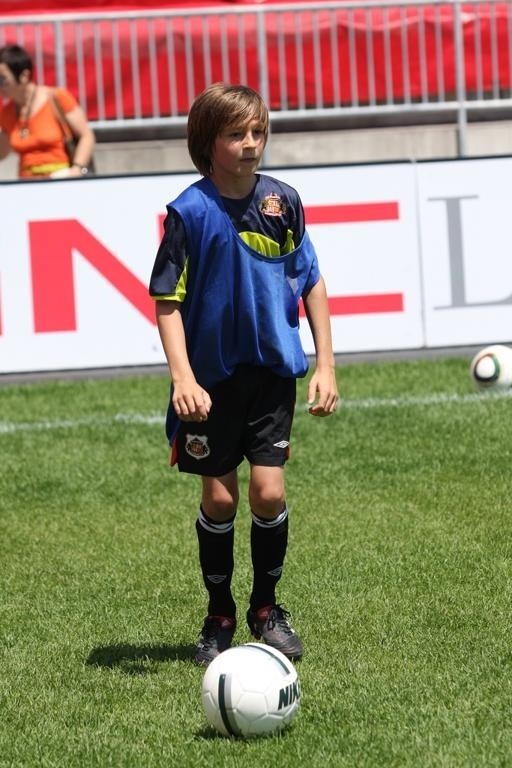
[
  {"left": 147, "top": 84, "right": 339, "bottom": 663},
  {"left": 0, "top": 45, "right": 97, "bottom": 183}
]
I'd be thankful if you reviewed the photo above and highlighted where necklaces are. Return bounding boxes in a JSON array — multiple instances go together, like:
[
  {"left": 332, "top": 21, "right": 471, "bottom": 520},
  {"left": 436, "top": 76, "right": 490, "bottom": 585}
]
[{"left": 15, "top": 86, "right": 36, "bottom": 138}]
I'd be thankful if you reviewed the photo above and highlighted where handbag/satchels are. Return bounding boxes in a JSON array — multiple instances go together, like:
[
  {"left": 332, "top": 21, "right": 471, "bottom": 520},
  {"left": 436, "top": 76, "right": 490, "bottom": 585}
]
[{"left": 65, "top": 136, "right": 97, "bottom": 176}]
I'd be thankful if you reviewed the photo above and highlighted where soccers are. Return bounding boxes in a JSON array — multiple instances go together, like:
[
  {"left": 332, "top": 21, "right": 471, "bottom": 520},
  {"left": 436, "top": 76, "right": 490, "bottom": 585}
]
[
  {"left": 469, "top": 344, "right": 512, "bottom": 391},
  {"left": 202, "top": 643, "right": 301, "bottom": 741}
]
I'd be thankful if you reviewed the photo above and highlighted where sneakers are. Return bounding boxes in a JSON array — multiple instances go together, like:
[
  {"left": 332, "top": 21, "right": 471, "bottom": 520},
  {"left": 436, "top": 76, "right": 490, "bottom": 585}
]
[
  {"left": 194, "top": 613, "right": 238, "bottom": 667},
  {"left": 244, "top": 606, "right": 305, "bottom": 661}
]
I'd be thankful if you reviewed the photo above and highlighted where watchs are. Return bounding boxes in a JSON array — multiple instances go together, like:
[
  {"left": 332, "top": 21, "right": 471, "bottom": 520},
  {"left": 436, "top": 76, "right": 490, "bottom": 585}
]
[{"left": 72, "top": 163, "right": 88, "bottom": 175}]
